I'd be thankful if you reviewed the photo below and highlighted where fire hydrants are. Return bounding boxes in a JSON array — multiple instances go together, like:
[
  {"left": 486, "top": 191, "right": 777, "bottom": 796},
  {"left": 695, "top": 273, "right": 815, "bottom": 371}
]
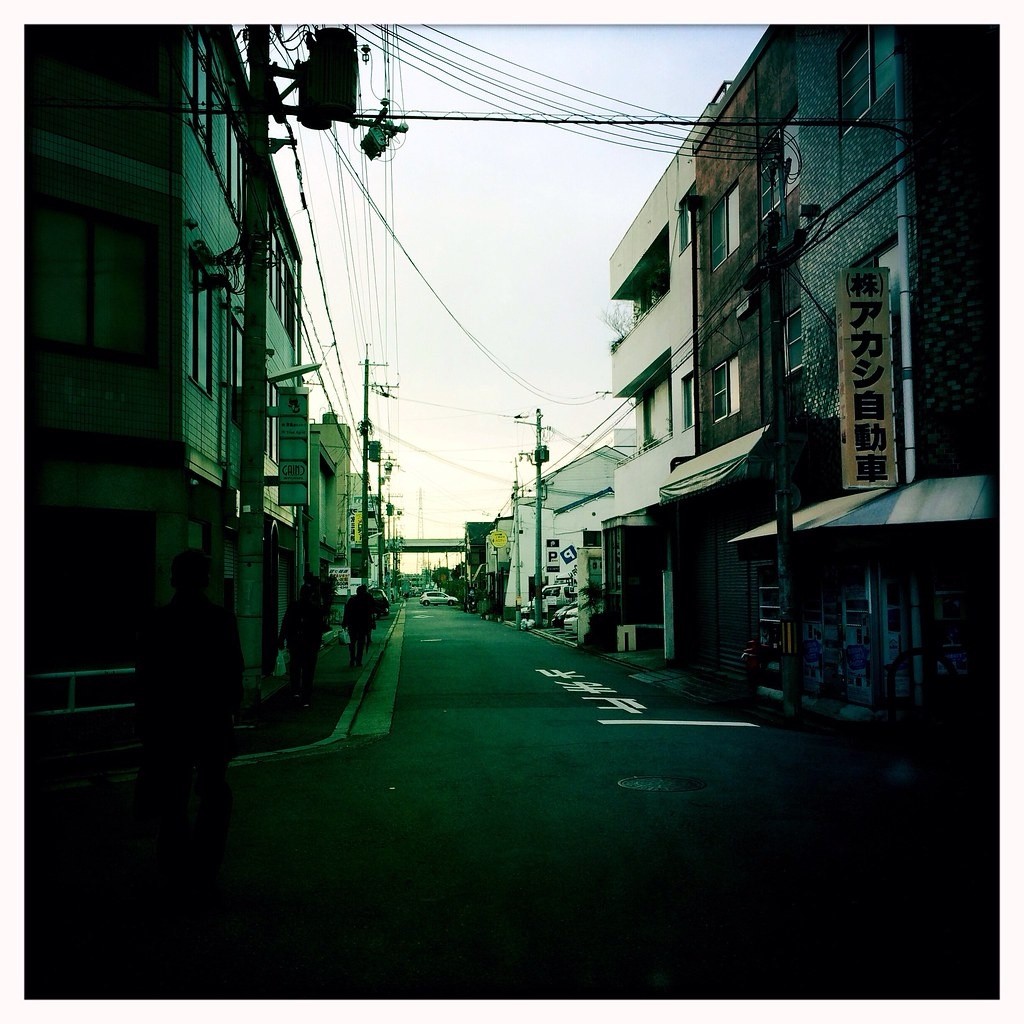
[{"left": 740, "top": 639, "right": 766, "bottom": 695}]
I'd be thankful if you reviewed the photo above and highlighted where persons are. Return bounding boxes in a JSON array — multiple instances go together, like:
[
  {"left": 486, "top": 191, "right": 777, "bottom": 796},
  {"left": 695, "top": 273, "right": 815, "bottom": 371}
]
[
  {"left": 343, "top": 584, "right": 375, "bottom": 667},
  {"left": 276, "top": 585, "right": 332, "bottom": 707},
  {"left": 133, "top": 549, "right": 246, "bottom": 898}
]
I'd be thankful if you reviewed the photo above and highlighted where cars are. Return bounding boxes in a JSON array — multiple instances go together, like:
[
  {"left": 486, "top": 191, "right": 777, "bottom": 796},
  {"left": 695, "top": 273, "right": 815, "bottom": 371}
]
[
  {"left": 552, "top": 600, "right": 578, "bottom": 629},
  {"left": 419, "top": 591, "right": 459, "bottom": 606},
  {"left": 564, "top": 608, "right": 578, "bottom": 635},
  {"left": 368, "top": 588, "right": 390, "bottom": 615}
]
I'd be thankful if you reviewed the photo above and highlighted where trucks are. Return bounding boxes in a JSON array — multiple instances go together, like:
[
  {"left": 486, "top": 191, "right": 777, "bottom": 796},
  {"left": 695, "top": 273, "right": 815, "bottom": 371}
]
[{"left": 529, "top": 583, "right": 578, "bottom": 614}]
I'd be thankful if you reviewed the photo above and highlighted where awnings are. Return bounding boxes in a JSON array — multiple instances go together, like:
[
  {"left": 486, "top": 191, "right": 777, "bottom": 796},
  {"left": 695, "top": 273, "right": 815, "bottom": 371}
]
[
  {"left": 726, "top": 474, "right": 998, "bottom": 545},
  {"left": 658, "top": 424, "right": 770, "bottom": 506}
]
